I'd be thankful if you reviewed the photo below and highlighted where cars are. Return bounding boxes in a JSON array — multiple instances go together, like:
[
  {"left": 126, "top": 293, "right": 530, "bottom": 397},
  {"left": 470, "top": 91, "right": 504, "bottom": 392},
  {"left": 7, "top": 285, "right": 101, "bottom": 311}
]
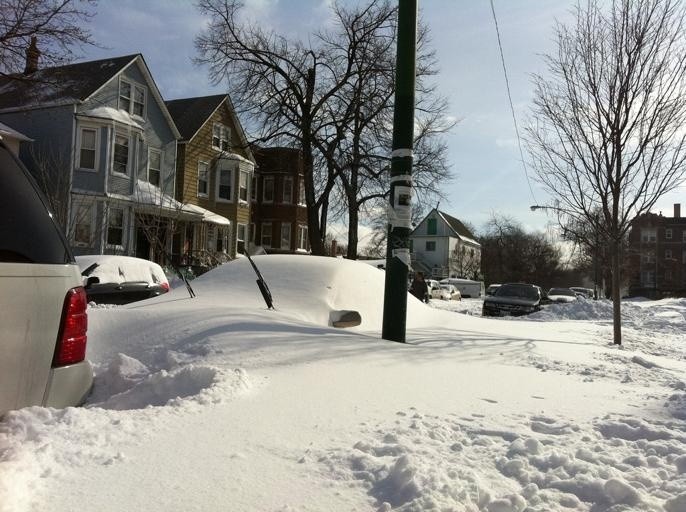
[
  {"left": 75, "top": 254, "right": 169, "bottom": 306},
  {"left": 0, "top": 124, "right": 96, "bottom": 414},
  {"left": 423, "top": 279, "right": 461, "bottom": 301},
  {"left": 482, "top": 282, "right": 546, "bottom": 317},
  {"left": 546, "top": 287, "right": 596, "bottom": 303}
]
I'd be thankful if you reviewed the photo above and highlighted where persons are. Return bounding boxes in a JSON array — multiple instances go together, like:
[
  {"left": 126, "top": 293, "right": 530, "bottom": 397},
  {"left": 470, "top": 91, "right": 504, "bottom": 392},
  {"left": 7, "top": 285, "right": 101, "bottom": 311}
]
[{"left": 410, "top": 271, "right": 430, "bottom": 306}]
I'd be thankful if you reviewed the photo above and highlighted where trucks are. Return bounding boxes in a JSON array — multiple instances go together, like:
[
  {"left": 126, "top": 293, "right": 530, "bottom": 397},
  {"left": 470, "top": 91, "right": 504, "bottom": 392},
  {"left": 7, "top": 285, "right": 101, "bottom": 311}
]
[{"left": 439, "top": 278, "right": 484, "bottom": 298}]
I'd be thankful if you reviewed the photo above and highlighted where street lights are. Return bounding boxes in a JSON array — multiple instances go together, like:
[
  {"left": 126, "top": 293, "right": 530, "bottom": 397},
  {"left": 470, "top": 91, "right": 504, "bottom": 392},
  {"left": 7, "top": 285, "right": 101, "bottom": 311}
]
[{"left": 530, "top": 206, "right": 598, "bottom": 300}]
